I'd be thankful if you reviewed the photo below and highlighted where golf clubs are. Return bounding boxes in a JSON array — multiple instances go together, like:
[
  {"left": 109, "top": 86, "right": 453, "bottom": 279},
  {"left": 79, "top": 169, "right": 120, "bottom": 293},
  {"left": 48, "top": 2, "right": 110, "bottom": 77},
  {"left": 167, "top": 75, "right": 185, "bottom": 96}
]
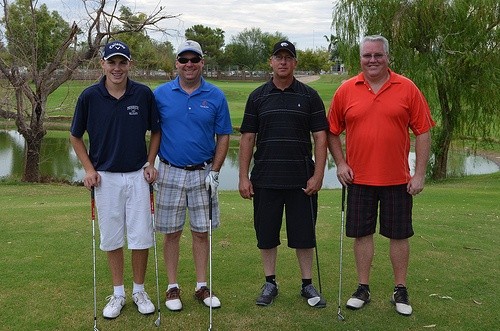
[
  {"left": 307, "top": 195, "right": 323, "bottom": 307},
  {"left": 89, "top": 185, "right": 100, "bottom": 331},
  {"left": 148, "top": 182, "right": 162, "bottom": 326},
  {"left": 207, "top": 184, "right": 213, "bottom": 331},
  {"left": 336, "top": 181, "right": 348, "bottom": 321}
]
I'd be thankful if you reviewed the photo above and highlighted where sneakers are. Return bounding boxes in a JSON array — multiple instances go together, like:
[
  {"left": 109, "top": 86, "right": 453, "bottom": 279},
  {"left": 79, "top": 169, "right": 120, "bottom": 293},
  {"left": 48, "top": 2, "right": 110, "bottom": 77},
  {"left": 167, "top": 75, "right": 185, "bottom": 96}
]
[
  {"left": 132, "top": 290, "right": 155, "bottom": 315},
  {"left": 102, "top": 292, "right": 126, "bottom": 319},
  {"left": 346, "top": 285, "right": 370, "bottom": 310},
  {"left": 164, "top": 286, "right": 183, "bottom": 311},
  {"left": 300, "top": 284, "right": 326, "bottom": 308},
  {"left": 255, "top": 282, "right": 278, "bottom": 306},
  {"left": 194, "top": 286, "right": 221, "bottom": 309},
  {"left": 390, "top": 283, "right": 413, "bottom": 315}
]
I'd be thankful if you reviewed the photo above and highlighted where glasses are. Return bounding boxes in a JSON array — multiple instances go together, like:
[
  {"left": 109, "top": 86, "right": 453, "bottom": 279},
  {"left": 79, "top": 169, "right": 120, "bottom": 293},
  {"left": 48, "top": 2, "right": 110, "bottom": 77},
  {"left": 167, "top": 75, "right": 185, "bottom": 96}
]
[
  {"left": 177, "top": 56, "right": 202, "bottom": 64},
  {"left": 361, "top": 53, "right": 386, "bottom": 59}
]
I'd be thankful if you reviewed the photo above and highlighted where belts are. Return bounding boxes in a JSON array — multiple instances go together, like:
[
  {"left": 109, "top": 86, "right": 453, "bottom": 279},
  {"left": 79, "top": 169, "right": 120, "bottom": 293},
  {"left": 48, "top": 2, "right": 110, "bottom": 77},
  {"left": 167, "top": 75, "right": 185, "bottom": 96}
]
[{"left": 159, "top": 157, "right": 213, "bottom": 171}]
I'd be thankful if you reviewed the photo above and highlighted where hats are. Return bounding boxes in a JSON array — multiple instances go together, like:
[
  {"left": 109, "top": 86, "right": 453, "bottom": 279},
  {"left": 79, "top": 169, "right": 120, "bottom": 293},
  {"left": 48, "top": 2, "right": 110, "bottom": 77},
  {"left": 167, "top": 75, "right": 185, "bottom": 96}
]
[
  {"left": 176, "top": 39, "right": 203, "bottom": 59},
  {"left": 102, "top": 40, "right": 132, "bottom": 62},
  {"left": 273, "top": 40, "right": 296, "bottom": 57}
]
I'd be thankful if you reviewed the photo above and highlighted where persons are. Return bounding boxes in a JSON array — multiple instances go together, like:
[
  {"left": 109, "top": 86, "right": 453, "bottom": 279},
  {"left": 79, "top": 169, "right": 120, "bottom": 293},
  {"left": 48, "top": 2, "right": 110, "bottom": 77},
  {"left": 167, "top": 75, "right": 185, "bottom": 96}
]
[
  {"left": 238, "top": 41, "right": 327, "bottom": 308},
  {"left": 153, "top": 40, "right": 233, "bottom": 312},
  {"left": 70, "top": 40, "right": 159, "bottom": 318},
  {"left": 326, "top": 34, "right": 435, "bottom": 315}
]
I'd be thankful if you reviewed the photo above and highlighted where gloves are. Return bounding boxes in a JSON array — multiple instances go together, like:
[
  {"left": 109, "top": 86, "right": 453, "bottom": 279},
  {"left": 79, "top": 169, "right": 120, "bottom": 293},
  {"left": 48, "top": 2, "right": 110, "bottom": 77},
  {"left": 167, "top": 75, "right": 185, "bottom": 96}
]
[{"left": 204, "top": 171, "right": 219, "bottom": 199}]
